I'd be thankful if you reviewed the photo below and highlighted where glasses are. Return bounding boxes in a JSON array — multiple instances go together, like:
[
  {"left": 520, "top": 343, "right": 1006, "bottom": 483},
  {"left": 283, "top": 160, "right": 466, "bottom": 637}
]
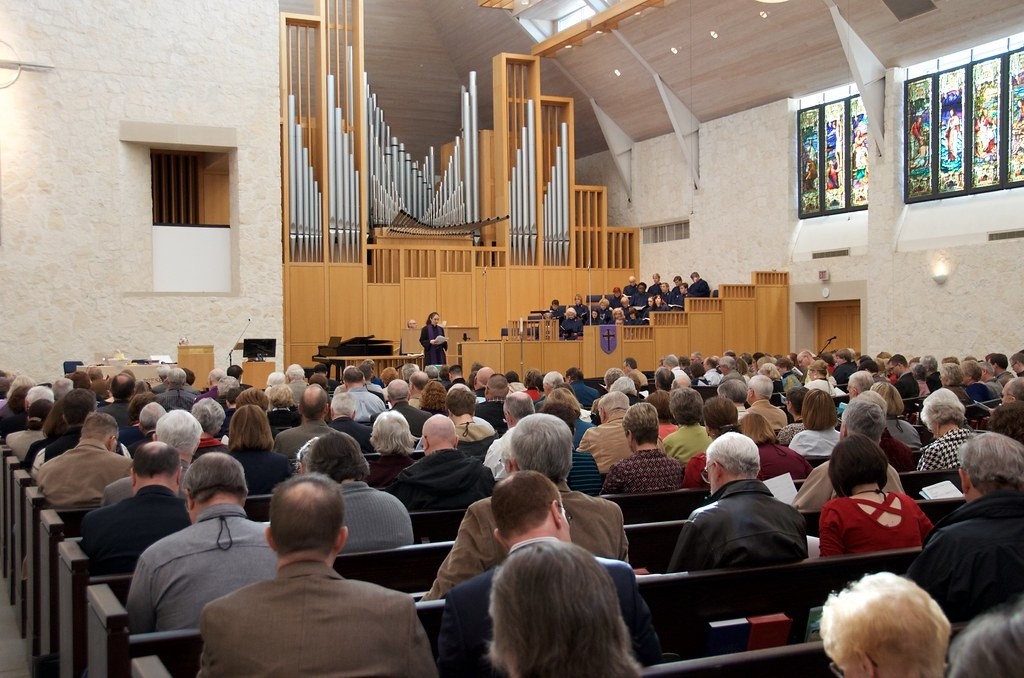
[
  {"left": 890, "top": 363, "right": 903, "bottom": 371},
  {"left": 557, "top": 504, "right": 573, "bottom": 523},
  {"left": 828, "top": 654, "right": 878, "bottom": 678},
  {"left": 700, "top": 461, "right": 725, "bottom": 484},
  {"left": 1011, "top": 362, "right": 1017, "bottom": 368}
]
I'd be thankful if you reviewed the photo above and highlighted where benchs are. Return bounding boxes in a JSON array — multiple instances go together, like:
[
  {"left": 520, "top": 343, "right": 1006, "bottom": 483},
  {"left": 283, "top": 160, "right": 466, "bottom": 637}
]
[{"left": 0, "top": 440, "right": 963, "bottom": 678}]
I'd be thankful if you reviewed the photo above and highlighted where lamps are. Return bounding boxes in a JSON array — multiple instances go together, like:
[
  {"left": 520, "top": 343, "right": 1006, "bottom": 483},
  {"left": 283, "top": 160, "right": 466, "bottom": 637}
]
[{"left": 932, "top": 275, "right": 946, "bottom": 283}]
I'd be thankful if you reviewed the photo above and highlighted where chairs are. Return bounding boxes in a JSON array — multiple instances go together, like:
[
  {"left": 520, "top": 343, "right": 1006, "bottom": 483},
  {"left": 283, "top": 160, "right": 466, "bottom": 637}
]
[{"left": 64, "top": 362, "right": 83, "bottom": 375}]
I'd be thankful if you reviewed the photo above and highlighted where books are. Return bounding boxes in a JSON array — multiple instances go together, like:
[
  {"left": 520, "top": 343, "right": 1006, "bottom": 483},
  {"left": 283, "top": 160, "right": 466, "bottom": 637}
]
[{"left": 919, "top": 481, "right": 963, "bottom": 500}]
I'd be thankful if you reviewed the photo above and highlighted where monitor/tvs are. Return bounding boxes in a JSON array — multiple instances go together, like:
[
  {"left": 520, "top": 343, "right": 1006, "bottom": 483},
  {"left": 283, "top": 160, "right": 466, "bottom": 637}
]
[{"left": 243, "top": 339, "right": 276, "bottom": 362}]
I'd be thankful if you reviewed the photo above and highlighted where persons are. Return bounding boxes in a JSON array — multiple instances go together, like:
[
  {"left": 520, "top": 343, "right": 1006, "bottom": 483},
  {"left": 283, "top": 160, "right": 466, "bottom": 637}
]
[
  {"left": 0, "top": 348, "right": 1024, "bottom": 678},
  {"left": 419, "top": 312, "right": 448, "bottom": 368},
  {"left": 399, "top": 320, "right": 418, "bottom": 355},
  {"left": 542, "top": 271, "right": 710, "bottom": 341}
]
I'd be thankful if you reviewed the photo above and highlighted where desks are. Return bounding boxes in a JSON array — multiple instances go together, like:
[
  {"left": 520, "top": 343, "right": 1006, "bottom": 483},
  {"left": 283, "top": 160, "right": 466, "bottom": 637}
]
[
  {"left": 327, "top": 355, "right": 425, "bottom": 379},
  {"left": 76, "top": 363, "right": 178, "bottom": 388}
]
